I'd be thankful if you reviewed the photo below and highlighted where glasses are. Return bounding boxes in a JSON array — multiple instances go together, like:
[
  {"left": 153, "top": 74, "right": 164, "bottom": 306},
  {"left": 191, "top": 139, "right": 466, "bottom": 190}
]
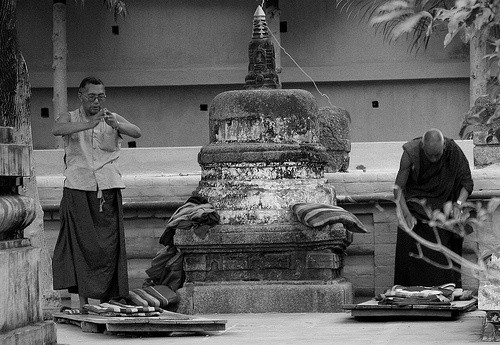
[{"left": 78, "top": 91, "right": 106, "bottom": 102}]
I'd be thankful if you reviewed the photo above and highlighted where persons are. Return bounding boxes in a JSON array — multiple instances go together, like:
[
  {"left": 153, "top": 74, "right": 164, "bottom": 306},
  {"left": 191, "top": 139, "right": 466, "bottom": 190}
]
[
  {"left": 390, "top": 129, "right": 474, "bottom": 289},
  {"left": 47, "top": 74, "right": 143, "bottom": 310}
]
